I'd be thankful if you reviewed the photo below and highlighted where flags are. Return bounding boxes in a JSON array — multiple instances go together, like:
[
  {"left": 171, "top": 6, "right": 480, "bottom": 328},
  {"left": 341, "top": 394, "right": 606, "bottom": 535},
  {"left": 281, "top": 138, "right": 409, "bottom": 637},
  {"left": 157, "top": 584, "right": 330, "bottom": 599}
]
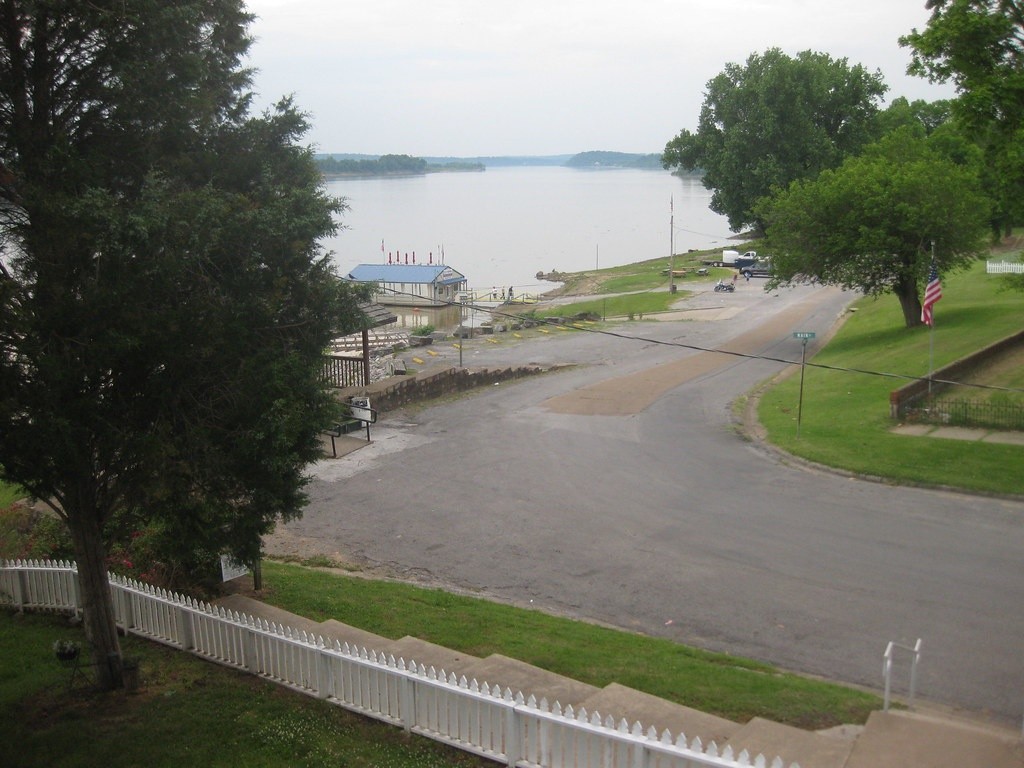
[{"left": 920, "top": 252, "right": 942, "bottom": 332}]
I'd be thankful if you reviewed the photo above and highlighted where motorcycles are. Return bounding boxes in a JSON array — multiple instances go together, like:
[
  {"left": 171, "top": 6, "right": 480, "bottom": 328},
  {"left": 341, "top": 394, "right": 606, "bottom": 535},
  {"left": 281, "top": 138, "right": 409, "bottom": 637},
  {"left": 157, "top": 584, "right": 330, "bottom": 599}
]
[{"left": 714, "top": 279, "right": 735, "bottom": 293}]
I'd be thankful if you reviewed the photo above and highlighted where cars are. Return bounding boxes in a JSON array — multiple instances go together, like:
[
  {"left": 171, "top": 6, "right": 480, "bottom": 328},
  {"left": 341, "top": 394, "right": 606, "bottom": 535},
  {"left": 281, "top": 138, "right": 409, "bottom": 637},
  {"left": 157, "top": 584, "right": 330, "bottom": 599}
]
[
  {"left": 740, "top": 260, "right": 778, "bottom": 278},
  {"left": 738, "top": 252, "right": 761, "bottom": 259}
]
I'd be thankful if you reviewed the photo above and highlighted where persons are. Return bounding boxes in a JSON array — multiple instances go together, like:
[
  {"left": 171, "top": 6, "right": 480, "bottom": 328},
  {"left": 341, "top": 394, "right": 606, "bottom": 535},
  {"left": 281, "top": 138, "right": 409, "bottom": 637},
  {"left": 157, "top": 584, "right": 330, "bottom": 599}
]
[
  {"left": 746, "top": 275, "right": 750, "bottom": 285},
  {"left": 492, "top": 286, "right": 513, "bottom": 301},
  {"left": 733, "top": 274, "right": 738, "bottom": 286}
]
